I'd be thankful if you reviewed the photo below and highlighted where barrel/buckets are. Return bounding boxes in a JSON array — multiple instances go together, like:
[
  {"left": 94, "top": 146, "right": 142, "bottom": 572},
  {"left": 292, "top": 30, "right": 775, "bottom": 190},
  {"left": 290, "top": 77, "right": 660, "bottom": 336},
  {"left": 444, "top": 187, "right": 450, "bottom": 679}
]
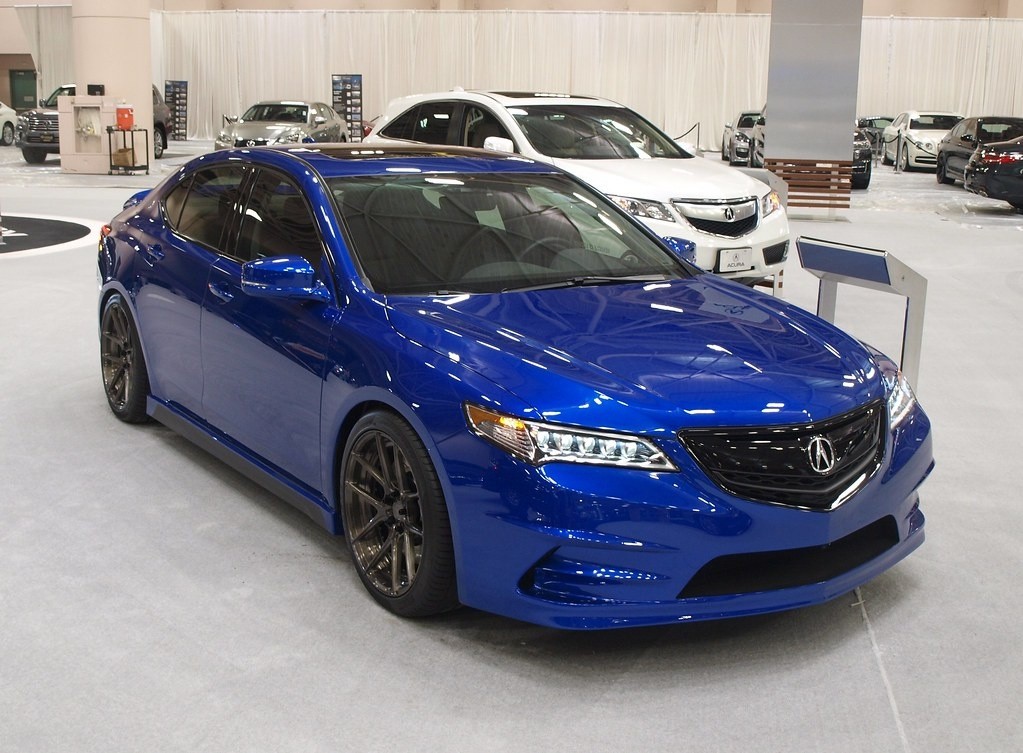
[{"left": 116, "top": 105, "right": 134, "bottom": 130}]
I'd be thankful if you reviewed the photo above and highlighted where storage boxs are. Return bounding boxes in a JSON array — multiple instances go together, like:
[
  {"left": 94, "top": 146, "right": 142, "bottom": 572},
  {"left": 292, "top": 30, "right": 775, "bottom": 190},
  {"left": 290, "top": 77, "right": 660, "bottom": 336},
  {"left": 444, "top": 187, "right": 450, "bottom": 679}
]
[{"left": 113, "top": 148, "right": 137, "bottom": 167}]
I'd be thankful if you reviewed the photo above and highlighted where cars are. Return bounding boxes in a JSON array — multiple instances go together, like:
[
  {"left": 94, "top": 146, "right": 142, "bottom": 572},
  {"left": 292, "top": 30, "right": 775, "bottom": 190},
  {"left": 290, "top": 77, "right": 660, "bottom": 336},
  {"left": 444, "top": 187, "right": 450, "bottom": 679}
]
[
  {"left": 0, "top": 101, "right": 18, "bottom": 147},
  {"left": 214, "top": 100, "right": 349, "bottom": 152},
  {"left": 96, "top": 141, "right": 934, "bottom": 630},
  {"left": 937, "top": 115, "right": 1023, "bottom": 210},
  {"left": 859, "top": 109, "right": 966, "bottom": 173},
  {"left": 361, "top": 86, "right": 789, "bottom": 287},
  {"left": 720, "top": 101, "right": 872, "bottom": 189}
]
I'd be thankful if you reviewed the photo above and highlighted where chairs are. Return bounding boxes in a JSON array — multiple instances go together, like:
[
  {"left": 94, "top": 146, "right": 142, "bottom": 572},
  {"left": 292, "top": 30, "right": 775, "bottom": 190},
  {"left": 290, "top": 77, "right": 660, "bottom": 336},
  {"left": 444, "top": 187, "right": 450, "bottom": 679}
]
[{"left": 472, "top": 124, "right": 581, "bottom": 157}]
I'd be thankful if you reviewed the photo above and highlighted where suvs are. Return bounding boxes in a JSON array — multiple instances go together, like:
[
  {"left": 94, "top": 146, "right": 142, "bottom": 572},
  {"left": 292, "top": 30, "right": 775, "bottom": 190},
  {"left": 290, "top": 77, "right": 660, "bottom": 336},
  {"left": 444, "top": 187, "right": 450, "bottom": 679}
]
[{"left": 16, "top": 81, "right": 173, "bottom": 163}]
